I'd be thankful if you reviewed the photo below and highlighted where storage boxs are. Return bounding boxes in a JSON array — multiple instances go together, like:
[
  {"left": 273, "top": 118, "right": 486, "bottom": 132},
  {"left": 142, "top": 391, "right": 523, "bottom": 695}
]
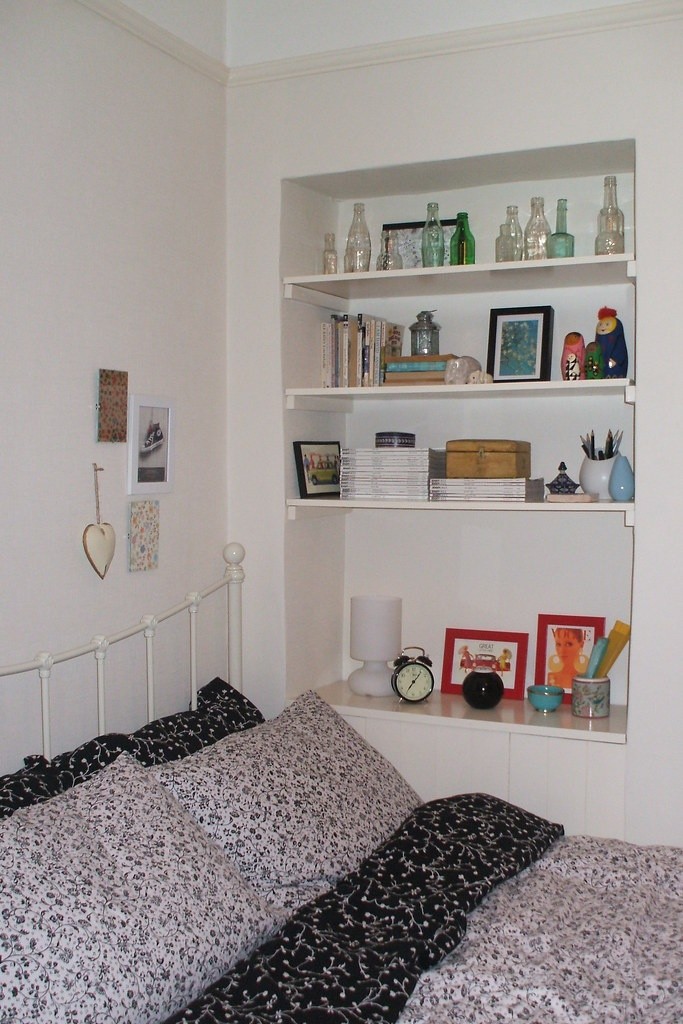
[{"left": 444, "top": 433, "right": 530, "bottom": 479}]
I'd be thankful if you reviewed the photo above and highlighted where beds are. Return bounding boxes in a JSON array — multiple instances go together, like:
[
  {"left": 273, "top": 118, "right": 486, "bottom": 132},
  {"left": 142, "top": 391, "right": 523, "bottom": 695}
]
[{"left": 0, "top": 541, "right": 683, "bottom": 1024}]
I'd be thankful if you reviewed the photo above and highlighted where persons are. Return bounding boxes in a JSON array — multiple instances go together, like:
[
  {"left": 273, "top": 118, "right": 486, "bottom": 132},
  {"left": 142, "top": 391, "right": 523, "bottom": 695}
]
[{"left": 546, "top": 628, "right": 585, "bottom": 689}]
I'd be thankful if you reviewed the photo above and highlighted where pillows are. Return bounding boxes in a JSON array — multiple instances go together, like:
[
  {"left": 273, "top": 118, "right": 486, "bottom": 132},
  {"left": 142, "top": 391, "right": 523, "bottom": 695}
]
[
  {"left": 146, "top": 686, "right": 423, "bottom": 924},
  {"left": 0, "top": 747, "right": 278, "bottom": 1024}
]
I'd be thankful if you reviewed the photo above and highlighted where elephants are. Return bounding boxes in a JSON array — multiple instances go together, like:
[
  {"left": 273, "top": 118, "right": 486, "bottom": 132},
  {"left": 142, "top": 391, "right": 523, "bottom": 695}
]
[{"left": 444, "top": 356, "right": 493, "bottom": 384}]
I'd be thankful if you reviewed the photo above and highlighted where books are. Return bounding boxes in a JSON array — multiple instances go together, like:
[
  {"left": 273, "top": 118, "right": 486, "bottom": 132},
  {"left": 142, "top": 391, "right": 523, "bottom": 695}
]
[
  {"left": 430, "top": 477, "right": 545, "bottom": 508},
  {"left": 547, "top": 493, "right": 600, "bottom": 503},
  {"left": 321, "top": 312, "right": 462, "bottom": 387},
  {"left": 339, "top": 448, "right": 446, "bottom": 501}
]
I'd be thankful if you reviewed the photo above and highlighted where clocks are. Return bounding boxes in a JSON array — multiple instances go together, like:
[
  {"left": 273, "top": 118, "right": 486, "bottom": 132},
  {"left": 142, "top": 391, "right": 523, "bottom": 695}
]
[{"left": 391, "top": 643, "right": 434, "bottom": 708}]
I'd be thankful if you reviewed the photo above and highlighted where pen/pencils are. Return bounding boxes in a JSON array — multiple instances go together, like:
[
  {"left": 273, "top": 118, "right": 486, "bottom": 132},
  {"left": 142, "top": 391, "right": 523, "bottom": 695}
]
[
  {"left": 598, "top": 450, "right": 604, "bottom": 460},
  {"left": 579, "top": 429, "right": 595, "bottom": 460},
  {"left": 606, "top": 429, "right": 623, "bottom": 459}
]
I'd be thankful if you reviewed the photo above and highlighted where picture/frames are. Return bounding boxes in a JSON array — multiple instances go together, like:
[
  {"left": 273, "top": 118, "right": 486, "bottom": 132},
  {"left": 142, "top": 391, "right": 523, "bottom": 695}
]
[
  {"left": 126, "top": 393, "right": 172, "bottom": 497},
  {"left": 535, "top": 613, "right": 607, "bottom": 707},
  {"left": 485, "top": 303, "right": 555, "bottom": 380},
  {"left": 443, "top": 625, "right": 528, "bottom": 702},
  {"left": 293, "top": 439, "right": 340, "bottom": 497}
]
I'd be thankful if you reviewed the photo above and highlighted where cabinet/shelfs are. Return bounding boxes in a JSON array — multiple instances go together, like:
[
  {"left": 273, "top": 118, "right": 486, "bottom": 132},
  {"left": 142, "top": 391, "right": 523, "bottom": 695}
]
[{"left": 283, "top": 253, "right": 638, "bottom": 742}]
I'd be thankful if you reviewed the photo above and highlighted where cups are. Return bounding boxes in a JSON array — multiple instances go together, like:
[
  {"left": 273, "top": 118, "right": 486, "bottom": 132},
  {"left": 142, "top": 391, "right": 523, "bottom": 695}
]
[
  {"left": 579, "top": 448, "right": 621, "bottom": 499},
  {"left": 571, "top": 675, "right": 610, "bottom": 718}
]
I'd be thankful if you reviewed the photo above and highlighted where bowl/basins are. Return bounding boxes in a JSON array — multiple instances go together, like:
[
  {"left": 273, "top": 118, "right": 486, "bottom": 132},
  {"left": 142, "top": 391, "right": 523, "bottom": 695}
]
[{"left": 527, "top": 684, "right": 564, "bottom": 712}]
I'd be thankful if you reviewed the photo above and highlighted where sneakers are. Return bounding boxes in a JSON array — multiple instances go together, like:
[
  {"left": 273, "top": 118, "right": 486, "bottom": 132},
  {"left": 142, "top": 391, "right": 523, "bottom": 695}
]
[{"left": 141, "top": 423, "right": 164, "bottom": 453}]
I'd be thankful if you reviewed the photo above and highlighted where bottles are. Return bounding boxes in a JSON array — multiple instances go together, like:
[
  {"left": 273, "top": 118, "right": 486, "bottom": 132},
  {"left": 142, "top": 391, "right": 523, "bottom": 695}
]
[
  {"left": 450, "top": 212, "right": 475, "bottom": 266},
  {"left": 376, "top": 230, "right": 403, "bottom": 271},
  {"left": 597, "top": 176, "right": 625, "bottom": 233},
  {"left": 596, "top": 203, "right": 624, "bottom": 255},
  {"left": 551, "top": 198, "right": 574, "bottom": 257},
  {"left": 409, "top": 309, "right": 441, "bottom": 355},
  {"left": 524, "top": 197, "right": 551, "bottom": 260},
  {"left": 422, "top": 203, "right": 444, "bottom": 267},
  {"left": 462, "top": 667, "right": 504, "bottom": 709},
  {"left": 506, "top": 206, "right": 522, "bottom": 260},
  {"left": 608, "top": 456, "right": 634, "bottom": 501},
  {"left": 323, "top": 233, "right": 337, "bottom": 274},
  {"left": 347, "top": 203, "right": 371, "bottom": 272},
  {"left": 495, "top": 224, "right": 515, "bottom": 263}
]
